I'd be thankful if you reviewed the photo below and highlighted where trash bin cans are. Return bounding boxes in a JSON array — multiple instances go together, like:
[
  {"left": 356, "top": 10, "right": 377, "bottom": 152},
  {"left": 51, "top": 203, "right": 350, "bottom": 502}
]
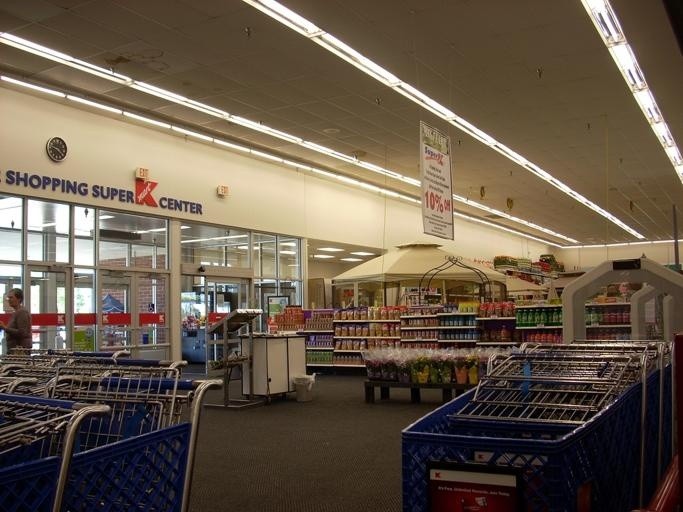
[
  {"left": 296, "top": 378, "right": 312, "bottom": 403},
  {"left": 227, "top": 360, "right": 242, "bottom": 380}
]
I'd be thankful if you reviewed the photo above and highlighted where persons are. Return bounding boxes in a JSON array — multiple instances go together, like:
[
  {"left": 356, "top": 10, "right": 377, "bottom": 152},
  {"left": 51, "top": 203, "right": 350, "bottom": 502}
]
[{"left": 0, "top": 288, "right": 32, "bottom": 373}]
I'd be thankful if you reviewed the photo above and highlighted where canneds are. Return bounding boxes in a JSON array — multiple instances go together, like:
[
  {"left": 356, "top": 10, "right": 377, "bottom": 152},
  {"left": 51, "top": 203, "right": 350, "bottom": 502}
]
[
  {"left": 274, "top": 305, "right": 305, "bottom": 323},
  {"left": 269, "top": 324, "right": 278, "bottom": 334}
]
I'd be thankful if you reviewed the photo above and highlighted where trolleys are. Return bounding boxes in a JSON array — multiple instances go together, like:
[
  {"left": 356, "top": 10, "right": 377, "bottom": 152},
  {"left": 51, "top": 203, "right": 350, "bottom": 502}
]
[
  {"left": 402, "top": 338, "right": 678, "bottom": 512},
  {"left": 1, "top": 341, "right": 229, "bottom": 511}
]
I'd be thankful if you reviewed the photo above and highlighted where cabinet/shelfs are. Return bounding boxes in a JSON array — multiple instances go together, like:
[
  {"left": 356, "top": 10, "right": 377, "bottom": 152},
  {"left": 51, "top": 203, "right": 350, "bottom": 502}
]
[{"left": 240, "top": 332, "right": 308, "bottom": 397}]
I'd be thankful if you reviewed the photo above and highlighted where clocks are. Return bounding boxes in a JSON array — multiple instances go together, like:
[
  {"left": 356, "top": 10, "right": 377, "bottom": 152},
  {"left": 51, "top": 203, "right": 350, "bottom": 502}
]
[{"left": 46, "top": 135, "right": 68, "bottom": 163}]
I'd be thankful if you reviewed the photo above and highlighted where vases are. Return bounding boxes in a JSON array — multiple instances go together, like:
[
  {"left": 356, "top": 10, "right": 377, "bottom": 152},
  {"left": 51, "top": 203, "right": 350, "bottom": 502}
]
[{"left": 366, "top": 369, "right": 491, "bottom": 385}]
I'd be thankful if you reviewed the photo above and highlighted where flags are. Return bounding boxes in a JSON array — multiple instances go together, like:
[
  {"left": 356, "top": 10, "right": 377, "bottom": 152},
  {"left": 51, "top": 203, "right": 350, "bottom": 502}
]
[{"left": 418, "top": 120, "right": 454, "bottom": 241}]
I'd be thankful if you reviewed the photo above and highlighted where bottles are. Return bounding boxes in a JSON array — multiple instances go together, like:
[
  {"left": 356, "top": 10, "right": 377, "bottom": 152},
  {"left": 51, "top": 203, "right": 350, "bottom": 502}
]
[
  {"left": 283, "top": 304, "right": 302, "bottom": 313},
  {"left": 482, "top": 325, "right": 516, "bottom": 342},
  {"left": 516, "top": 307, "right": 633, "bottom": 342}
]
